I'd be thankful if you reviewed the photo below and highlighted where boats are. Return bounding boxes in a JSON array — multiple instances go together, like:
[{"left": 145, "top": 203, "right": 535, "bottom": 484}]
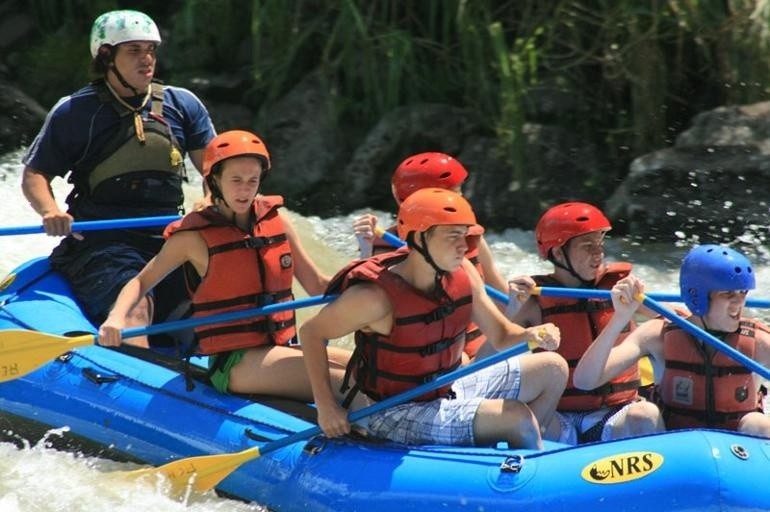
[{"left": 1, "top": 256, "right": 768, "bottom": 512}]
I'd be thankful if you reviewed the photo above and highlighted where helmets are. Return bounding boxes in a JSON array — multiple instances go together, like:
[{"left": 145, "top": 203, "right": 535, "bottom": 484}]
[
  {"left": 680, "top": 243, "right": 756, "bottom": 317},
  {"left": 397, "top": 188, "right": 477, "bottom": 241},
  {"left": 536, "top": 202, "right": 613, "bottom": 261},
  {"left": 203, "top": 130, "right": 271, "bottom": 178},
  {"left": 392, "top": 153, "right": 468, "bottom": 204},
  {"left": 90, "top": 9, "right": 162, "bottom": 59}
]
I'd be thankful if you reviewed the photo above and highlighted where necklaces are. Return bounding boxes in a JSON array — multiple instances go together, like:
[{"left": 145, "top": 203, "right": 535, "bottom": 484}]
[{"left": 106, "top": 79, "right": 152, "bottom": 143}]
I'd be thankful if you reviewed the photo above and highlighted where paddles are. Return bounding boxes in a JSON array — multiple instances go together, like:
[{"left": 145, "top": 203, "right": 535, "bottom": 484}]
[
  {"left": 0, "top": 293, "right": 344, "bottom": 383},
  {"left": 125, "top": 330, "right": 547, "bottom": 493}
]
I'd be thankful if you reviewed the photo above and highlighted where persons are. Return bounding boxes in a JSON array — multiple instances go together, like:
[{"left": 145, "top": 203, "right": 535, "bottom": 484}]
[
  {"left": 352, "top": 154, "right": 509, "bottom": 366},
  {"left": 300, "top": 187, "right": 569, "bottom": 450},
  {"left": 97, "top": 129, "right": 376, "bottom": 438},
  {"left": 474, "top": 202, "right": 678, "bottom": 441},
  {"left": 20, "top": 10, "right": 218, "bottom": 353},
  {"left": 573, "top": 245, "right": 770, "bottom": 440}
]
